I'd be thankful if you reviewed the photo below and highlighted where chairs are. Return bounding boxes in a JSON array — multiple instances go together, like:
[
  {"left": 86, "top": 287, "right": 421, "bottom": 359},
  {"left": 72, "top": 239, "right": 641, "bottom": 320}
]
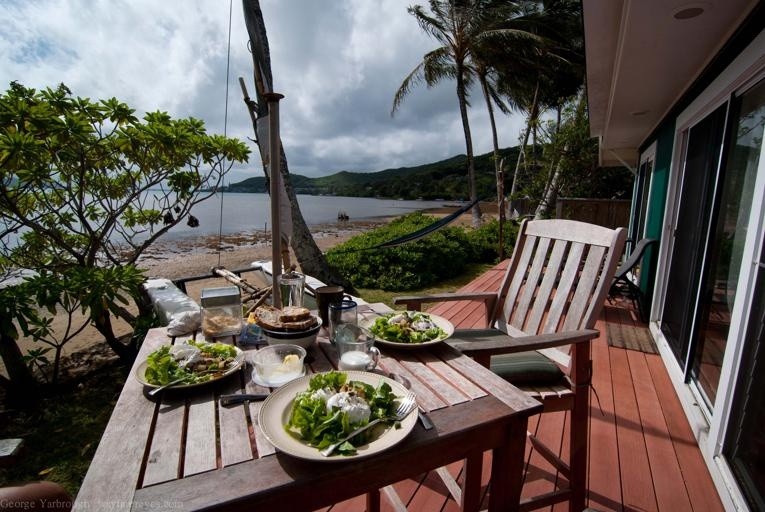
[
  {"left": 530, "top": 217, "right": 658, "bottom": 320},
  {"left": 395, "top": 215, "right": 629, "bottom": 509}
]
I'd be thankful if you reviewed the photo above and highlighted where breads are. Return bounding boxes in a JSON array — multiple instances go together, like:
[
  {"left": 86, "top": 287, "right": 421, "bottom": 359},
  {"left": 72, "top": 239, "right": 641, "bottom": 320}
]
[{"left": 252, "top": 303, "right": 317, "bottom": 329}]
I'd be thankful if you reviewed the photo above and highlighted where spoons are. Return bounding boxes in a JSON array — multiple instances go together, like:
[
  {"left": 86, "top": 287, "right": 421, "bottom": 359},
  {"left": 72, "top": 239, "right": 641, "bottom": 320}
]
[{"left": 388, "top": 373, "right": 436, "bottom": 432}]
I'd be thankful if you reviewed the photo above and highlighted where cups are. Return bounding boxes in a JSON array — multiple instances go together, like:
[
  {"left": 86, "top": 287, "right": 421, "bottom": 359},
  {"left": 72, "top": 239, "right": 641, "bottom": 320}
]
[
  {"left": 252, "top": 345, "right": 306, "bottom": 384},
  {"left": 279, "top": 273, "right": 306, "bottom": 308},
  {"left": 315, "top": 284, "right": 381, "bottom": 372}
]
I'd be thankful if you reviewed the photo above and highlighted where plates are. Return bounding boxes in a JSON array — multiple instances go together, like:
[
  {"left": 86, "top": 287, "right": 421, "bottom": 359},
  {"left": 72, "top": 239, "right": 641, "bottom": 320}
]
[
  {"left": 360, "top": 311, "right": 455, "bottom": 349},
  {"left": 257, "top": 314, "right": 322, "bottom": 337},
  {"left": 258, "top": 370, "right": 419, "bottom": 463},
  {"left": 135, "top": 342, "right": 245, "bottom": 391},
  {"left": 248, "top": 368, "right": 306, "bottom": 388}
]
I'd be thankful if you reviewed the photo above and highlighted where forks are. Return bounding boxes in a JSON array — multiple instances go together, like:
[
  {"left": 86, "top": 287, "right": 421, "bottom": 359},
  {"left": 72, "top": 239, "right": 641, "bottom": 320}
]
[
  {"left": 149, "top": 361, "right": 246, "bottom": 395},
  {"left": 319, "top": 393, "right": 419, "bottom": 457}
]
